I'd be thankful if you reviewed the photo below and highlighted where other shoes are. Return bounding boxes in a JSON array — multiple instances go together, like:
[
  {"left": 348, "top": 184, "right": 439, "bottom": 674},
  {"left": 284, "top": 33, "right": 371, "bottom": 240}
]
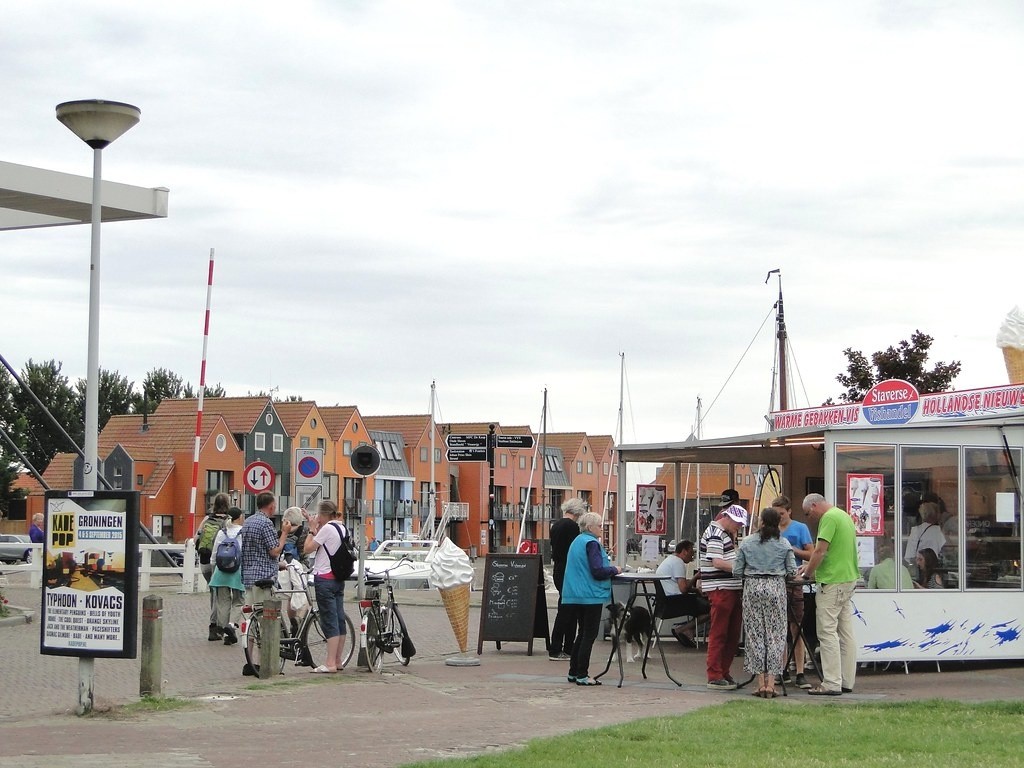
[
  {"left": 208, "top": 625, "right": 222, "bottom": 640},
  {"left": 243, "top": 663, "right": 261, "bottom": 677},
  {"left": 224, "top": 624, "right": 238, "bottom": 643}
]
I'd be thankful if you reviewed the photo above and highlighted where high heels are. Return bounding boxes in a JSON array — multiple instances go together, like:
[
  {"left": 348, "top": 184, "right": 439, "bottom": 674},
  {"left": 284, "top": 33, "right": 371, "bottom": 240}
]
[
  {"left": 752, "top": 685, "right": 765, "bottom": 697},
  {"left": 765, "top": 685, "right": 780, "bottom": 699}
]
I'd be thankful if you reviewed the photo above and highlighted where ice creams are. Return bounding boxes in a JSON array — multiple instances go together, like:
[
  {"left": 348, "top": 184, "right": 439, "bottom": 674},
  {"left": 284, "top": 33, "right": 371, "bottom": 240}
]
[
  {"left": 851, "top": 480, "right": 879, "bottom": 506},
  {"left": 640, "top": 489, "right": 662, "bottom": 510},
  {"left": 997, "top": 304, "right": 1024, "bottom": 384},
  {"left": 430, "top": 537, "right": 474, "bottom": 653}
]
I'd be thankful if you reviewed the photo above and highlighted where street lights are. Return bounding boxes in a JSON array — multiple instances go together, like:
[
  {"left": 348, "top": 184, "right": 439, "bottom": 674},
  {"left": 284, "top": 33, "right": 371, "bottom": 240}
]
[{"left": 55, "top": 99, "right": 143, "bottom": 714}]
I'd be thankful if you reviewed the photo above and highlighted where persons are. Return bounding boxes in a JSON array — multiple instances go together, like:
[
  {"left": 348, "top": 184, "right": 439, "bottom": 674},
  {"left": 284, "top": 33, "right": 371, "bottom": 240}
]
[
  {"left": 655, "top": 540, "right": 711, "bottom": 648},
  {"left": 731, "top": 508, "right": 798, "bottom": 699},
  {"left": 700, "top": 504, "right": 748, "bottom": 690},
  {"left": 549, "top": 498, "right": 588, "bottom": 661},
  {"left": 194, "top": 493, "right": 246, "bottom": 645},
  {"left": 802, "top": 493, "right": 861, "bottom": 696},
  {"left": 304, "top": 500, "right": 350, "bottom": 673},
  {"left": 356, "top": 532, "right": 379, "bottom": 551},
  {"left": 868, "top": 546, "right": 914, "bottom": 589},
  {"left": 277, "top": 506, "right": 318, "bottom": 667},
  {"left": 902, "top": 487, "right": 980, "bottom": 566},
  {"left": 713, "top": 489, "right": 739, "bottom": 550},
  {"left": 561, "top": 512, "right": 622, "bottom": 685},
  {"left": 772, "top": 496, "right": 814, "bottom": 689},
  {"left": 912, "top": 548, "right": 945, "bottom": 589},
  {"left": 29, "top": 513, "right": 45, "bottom": 543},
  {"left": 240, "top": 490, "right": 292, "bottom": 676}
]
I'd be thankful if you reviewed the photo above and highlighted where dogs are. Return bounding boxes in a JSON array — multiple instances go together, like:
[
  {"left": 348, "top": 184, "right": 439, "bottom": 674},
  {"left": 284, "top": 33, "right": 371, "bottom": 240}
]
[{"left": 605, "top": 601, "right": 654, "bottom": 663}]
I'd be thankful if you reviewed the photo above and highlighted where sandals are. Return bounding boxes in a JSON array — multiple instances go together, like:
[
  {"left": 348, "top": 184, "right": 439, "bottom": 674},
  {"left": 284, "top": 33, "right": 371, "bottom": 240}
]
[
  {"left": 808, "top": 685, "right": 842, "bottom": 695},
  {"left": 577, "top": 676, "right": 602, "bottom": 685},
  {"left": 567, "top": 675, "right": 576, "bottom": 682},
  {"left": 842, "top": 687, "right": 852, "bottom": 692}
]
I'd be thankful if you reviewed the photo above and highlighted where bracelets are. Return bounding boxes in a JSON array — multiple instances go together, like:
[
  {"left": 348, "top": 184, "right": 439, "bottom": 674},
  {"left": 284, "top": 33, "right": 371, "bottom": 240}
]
[
  {"left": 309, "top": 530, "right": 316, "bottom": 536},
  {"left": 801, "top": 573, "right": 810, "bottom": 580}
]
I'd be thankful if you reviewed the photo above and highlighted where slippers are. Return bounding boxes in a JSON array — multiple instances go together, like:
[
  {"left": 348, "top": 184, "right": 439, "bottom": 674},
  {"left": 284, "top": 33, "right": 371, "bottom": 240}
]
[
  {"left": 671, "top": 628, "right": 696, "bottom": 647},
  {"left": 338, "top": 669, "right": 343, "bottom": 670},
  {"left": 310, "top": 665, "right": 335, "bottom": 673}
]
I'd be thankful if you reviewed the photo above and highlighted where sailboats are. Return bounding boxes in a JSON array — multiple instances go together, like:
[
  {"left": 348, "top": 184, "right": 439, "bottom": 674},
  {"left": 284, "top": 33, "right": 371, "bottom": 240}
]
[
  {"left": 515, "top": 387, "right": 573, "bottom": 609},
  {"left": 350, "top": 381, "right": 479, "bottom": 592}
]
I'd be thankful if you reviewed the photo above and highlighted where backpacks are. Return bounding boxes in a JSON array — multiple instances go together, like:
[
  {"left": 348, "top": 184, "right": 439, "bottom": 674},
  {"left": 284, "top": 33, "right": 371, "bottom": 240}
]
[
  {"left": 197, "top": 517, "right": 229, "bottom": 564},
  {"left": 216, "top": 528, "right": 241, "bottom": 572},
  {"left": 323, "top": 523, "right": 357, "bottom": 580}
]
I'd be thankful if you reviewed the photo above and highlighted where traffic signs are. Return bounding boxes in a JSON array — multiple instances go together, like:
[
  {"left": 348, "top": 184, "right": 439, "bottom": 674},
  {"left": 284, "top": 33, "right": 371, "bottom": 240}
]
[
  {"left": 445, "top": 447, "right": 487, "bottom": 462},
  {"left": 496, "top": 434, "right": 536, "bottom": 449},
  {"left": 444, "top": 434, "right": 488, "bottom": 448}
]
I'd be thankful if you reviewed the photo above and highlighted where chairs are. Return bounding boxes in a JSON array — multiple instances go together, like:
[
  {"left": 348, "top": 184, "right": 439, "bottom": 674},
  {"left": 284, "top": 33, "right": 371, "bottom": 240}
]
[{"left": 652, "top": 580, "right": 706, "bottom": 649}]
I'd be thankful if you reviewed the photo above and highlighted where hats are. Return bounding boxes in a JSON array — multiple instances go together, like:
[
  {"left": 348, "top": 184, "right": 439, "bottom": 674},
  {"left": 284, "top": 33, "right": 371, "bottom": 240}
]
[
  {"left": 722, "top": 505, "right": 748, "bottom": 527},
  {"left": 718, "top": 489, "right": 738, "bottom": 506}
]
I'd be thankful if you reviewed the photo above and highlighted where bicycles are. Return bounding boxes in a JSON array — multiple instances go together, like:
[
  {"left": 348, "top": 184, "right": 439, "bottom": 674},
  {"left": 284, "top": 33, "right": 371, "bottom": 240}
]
[
  {"left": 354, "top": 556, "right": 416, "bottom": 674},
  {"left": 240, "top": 563, "right": 356, "bottom": 678}
]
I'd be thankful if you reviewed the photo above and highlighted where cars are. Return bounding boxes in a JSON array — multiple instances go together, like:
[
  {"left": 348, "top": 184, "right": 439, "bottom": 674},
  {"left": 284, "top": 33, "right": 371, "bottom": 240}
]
[
  {"left": 0, "top": 534, "right": 33, "bottom": 565},
  {"left": 137, "top": 535, "right": 200, "bottom": 568}
]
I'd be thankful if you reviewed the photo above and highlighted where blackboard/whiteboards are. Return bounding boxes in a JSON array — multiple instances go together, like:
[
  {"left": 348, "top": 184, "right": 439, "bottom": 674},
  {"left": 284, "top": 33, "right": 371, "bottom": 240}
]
[{"left": 478, "top": 552, "right": 550, "bottom": 641}]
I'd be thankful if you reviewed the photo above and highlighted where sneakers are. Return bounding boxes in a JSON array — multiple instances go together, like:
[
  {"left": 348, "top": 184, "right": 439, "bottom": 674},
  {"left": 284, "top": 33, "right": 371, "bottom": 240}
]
[
  {"left": 727, "top": 676, "right": 738, "bottom": 684},
  {"left": 707, "top": 678, "right": 737, "bottom": 689},
  {"left": 774, "top": 671, "right": 792, "bottom": 684},
  {"left": 795, "top": 673, "right": 812, "bottom": 688},
  {"left": 549, "top": 651, "right": 571, "bottom": 661}
]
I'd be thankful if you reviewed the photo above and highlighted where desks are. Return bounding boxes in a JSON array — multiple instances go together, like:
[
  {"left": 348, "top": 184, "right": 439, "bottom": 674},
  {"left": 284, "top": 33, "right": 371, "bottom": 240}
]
[
  {"left": 737, "top": 581, "right": 824, "bottom": 696},
  {"left": 593, "top": 572, "right": 682, "bottom": 688}
]
